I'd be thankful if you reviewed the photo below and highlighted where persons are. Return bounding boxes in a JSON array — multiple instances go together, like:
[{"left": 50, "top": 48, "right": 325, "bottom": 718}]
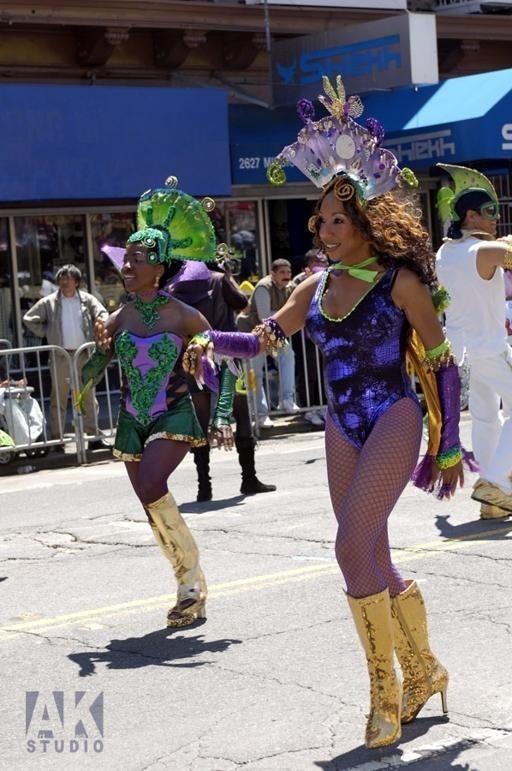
[
  {"left": 1, "top": 343, "right": 52, "bottom": 453},
  {"left": 22, "top": 265, "right": 113, "bottom": 452}
]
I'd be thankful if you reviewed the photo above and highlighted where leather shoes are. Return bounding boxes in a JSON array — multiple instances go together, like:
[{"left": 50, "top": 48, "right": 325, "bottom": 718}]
[{"left": 88, "top": 439, "right": 113, "bottom": 450}]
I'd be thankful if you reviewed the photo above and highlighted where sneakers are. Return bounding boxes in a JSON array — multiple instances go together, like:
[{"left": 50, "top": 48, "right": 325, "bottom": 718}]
[
  {"left": 259, "top": 415, "right": 273, "bottom": 428},
  {"left": 305, "top": 406, "right": 328, "bottom": 426},
  {"left": 277, "top": 402, "right": 301, "bottom": 414},
  {"left": 470, "top": 479, "right": 512, "bottom": 521}
]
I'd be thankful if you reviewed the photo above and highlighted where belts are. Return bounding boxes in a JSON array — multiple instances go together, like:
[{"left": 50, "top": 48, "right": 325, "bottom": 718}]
[{"left": 64, "top": 349, "right": 77, "bottom": 353}]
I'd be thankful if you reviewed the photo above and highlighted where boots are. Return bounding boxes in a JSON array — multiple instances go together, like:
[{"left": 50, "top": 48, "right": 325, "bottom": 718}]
[
  {"left": 235, "top": 431, "right": 276, "bottom": 492},
  {"left": 342, "top": 586, "right": 402, "bottom": 750},
  {"left": 190, "top": 444, "right": 212, "bottom": 502},
  {"left": 389, "top": 580, "right": 449, "bottom": 725},
  {"left": 142, "top": 492, "right": 209, "bottom": 630}
]
